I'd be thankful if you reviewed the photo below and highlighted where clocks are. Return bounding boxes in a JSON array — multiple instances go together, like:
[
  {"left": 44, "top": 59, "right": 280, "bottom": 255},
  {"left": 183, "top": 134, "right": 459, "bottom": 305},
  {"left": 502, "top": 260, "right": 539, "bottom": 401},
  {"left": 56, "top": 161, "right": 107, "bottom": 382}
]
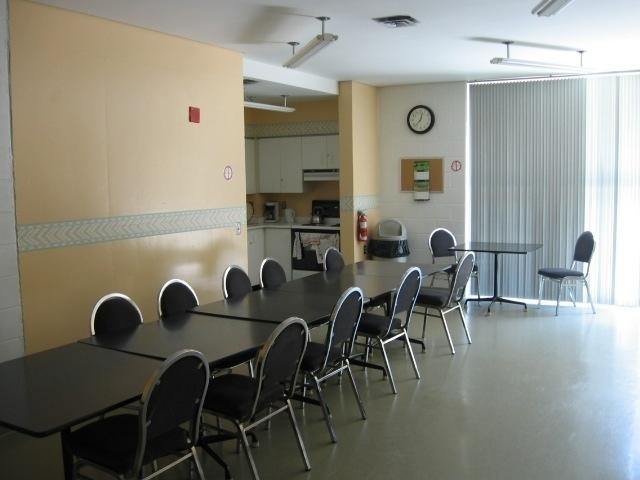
[{"left": 407, "top": 105, "right": 435, "bottom": 134}]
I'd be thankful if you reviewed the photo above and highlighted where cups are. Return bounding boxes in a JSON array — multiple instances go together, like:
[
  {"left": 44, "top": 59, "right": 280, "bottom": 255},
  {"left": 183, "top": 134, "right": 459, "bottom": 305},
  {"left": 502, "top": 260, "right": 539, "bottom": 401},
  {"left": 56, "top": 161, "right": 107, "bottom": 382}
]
[{"left": 285, "top": 208, "right": 295, "bottom": 223}]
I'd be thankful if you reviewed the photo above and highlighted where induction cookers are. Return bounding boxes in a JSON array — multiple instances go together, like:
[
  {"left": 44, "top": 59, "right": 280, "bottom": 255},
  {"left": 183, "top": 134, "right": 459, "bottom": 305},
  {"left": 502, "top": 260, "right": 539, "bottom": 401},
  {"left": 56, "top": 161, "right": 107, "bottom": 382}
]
[{"left": 293, "top": 223, "right": 340, "bottom": 231}]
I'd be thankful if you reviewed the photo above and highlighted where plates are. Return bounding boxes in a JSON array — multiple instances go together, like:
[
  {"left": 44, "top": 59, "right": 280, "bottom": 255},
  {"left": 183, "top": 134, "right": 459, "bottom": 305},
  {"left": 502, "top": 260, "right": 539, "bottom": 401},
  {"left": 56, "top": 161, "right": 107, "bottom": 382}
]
[{"left": 247, "top": 200, "right": 255, "bottom": 225}]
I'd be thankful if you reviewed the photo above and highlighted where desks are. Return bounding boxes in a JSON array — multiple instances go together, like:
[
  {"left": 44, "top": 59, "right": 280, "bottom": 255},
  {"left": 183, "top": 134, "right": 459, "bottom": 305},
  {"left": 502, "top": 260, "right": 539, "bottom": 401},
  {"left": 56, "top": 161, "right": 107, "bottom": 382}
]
[
  {"left": 0, "top": 341, "right": 165, "bottom": 480},
  {"left": 448, "top": 241, "right": 543, "bottom": 315}
]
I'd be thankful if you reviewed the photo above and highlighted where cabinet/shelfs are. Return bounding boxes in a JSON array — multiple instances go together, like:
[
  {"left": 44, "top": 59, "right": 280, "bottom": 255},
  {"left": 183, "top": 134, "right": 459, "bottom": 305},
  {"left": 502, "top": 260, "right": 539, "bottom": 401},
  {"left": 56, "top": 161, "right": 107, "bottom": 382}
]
[
  {"left": 259, "top": 137, "right": 313, "bottom": 193},
  {"left": 245, "top": 138, "right": 260, "bottom": 195},
  {"left": 302, "top": 135, "right": 339, "bottom": 169}
]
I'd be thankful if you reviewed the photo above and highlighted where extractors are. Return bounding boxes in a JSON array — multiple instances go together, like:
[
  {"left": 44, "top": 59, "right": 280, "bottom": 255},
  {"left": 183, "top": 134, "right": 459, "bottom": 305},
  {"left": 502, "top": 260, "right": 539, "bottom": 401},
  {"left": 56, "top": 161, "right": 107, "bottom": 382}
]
[{"left": 304, "top": 169, "right": 339, "bottom": 182}]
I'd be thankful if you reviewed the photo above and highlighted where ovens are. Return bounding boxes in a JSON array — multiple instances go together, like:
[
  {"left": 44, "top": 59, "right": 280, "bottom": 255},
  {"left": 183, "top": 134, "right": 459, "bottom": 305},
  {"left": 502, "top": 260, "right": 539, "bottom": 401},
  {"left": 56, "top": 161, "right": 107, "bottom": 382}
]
[{"left": 292, "top": 232, "right": 341, "bottom": 279}]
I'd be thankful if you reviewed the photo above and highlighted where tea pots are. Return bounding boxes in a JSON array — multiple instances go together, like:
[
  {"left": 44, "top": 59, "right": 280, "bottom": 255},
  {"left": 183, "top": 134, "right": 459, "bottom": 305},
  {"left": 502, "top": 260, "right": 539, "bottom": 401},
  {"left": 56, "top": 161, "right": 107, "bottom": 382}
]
[{"left": 310, "top": 206, "right": 324, "bottom": 223}]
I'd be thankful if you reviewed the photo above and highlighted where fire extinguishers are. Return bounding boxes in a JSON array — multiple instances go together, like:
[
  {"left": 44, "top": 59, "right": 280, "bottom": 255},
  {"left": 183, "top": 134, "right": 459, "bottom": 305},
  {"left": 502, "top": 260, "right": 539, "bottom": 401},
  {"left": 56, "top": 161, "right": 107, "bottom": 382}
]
[{"left": 357, "top": 211, "right": 368, "bottom": 241}]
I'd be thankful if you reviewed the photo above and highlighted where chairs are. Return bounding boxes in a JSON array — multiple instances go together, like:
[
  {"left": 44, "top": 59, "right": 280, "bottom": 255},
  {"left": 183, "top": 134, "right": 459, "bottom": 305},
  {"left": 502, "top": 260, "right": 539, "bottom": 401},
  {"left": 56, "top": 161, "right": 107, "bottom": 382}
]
[
  {"left": 537, "top": 231, "right": 596, "bottom": 316},
  {"left": 428, "top": 228, "right": 480, "bottom": 302}
]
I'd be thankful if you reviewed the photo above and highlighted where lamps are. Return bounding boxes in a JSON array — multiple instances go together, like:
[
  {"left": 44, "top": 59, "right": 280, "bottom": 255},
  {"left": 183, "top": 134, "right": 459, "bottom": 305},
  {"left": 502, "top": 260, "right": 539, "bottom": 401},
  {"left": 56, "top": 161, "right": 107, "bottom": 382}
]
[
  {"left": 282, "top": 16, "right": 338, "bottom": 70},
  {"left": 244, "top": 95, "right": 296, "bottom": 113},
  {"left": 490, "top": 42, "right": 587, "bottom": 75}
]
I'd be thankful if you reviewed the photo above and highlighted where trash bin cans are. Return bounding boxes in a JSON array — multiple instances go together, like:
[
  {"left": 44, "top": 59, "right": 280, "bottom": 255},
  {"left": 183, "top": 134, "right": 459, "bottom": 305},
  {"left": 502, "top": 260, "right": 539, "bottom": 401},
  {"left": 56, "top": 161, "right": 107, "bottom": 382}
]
[{"left": 371, "top": 219, "right": 407, "bottom": 263}]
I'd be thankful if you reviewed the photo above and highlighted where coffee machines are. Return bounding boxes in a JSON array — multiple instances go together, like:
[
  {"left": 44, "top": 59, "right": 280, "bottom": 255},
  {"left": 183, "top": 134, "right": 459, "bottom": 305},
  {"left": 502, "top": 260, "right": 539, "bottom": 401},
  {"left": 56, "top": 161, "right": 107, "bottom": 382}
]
[{"left": 264, "top": 201, "right": 281, "bottom": 223}]
